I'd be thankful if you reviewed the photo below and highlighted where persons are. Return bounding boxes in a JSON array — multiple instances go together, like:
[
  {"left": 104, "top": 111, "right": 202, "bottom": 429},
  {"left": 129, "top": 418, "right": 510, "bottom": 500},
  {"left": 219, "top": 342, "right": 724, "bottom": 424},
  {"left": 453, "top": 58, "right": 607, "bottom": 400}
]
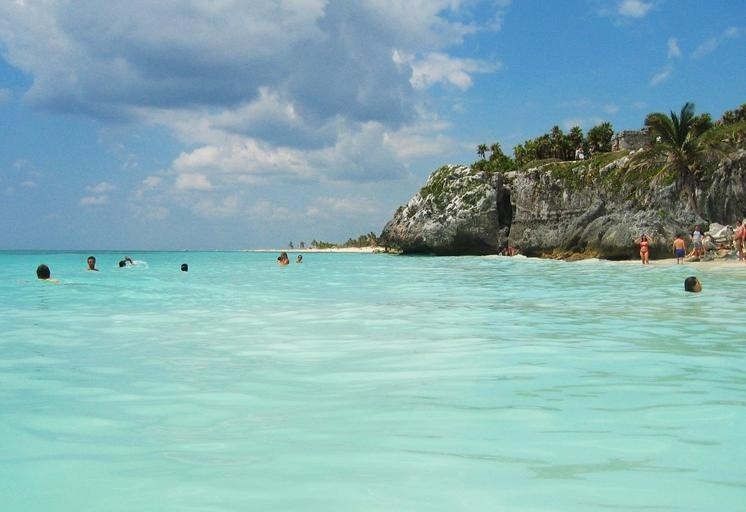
[
  {"left": 743, "top": 224, "right": 746, "bottom": 249},
  {"left": 37, "top": 265, "right": 58, "bottom": 283},
  {"left": 735, "top": 220, "right": 744, "bottom": 261},
  {"left": 634, "top": 234, "right": 652, "bottom": 264},
  {"left": 181, "top": 264, "right": 188, "bottom": 271},
  {"left": 692, "top": 225, "right": 705, "bottom": 257},
  {"left": 279, "top": 252, "right": 289, "bottom": 264},
  {"left": 685, "top": 277, "right": 701, "bottom": 292},
  {"left": 673, "top": 234, "right": 686, "bottom": 264},
  {"left": 119, "top": 256, "right": 133, "bottom": 267},
  {"left": 87, "top": 256, "right": 99, "bottom": 271},
  {"left": 296, "top": 255, "right": 303, "bottom": 263}
]
